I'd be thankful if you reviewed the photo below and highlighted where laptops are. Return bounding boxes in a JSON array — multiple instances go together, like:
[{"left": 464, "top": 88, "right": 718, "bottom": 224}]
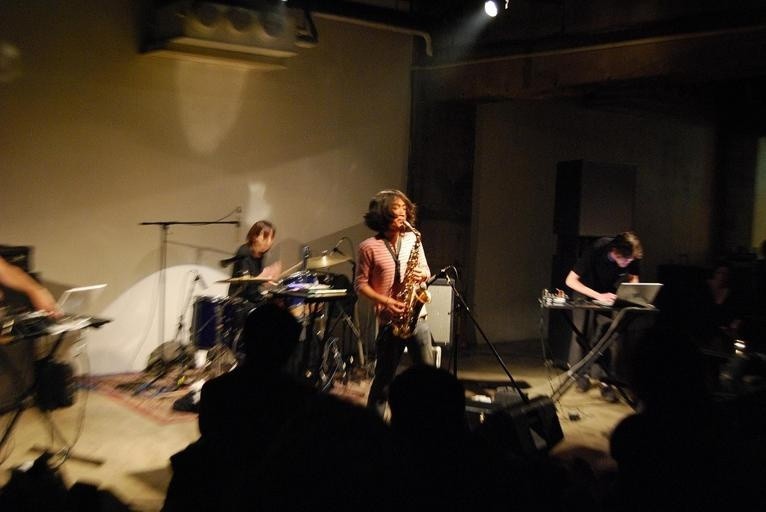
[
  {"left": 54, "top": 283, "right": 108, "bottom": 325},
  {"left": 591, "top": 282, "right": 664, "bottom": 308}
]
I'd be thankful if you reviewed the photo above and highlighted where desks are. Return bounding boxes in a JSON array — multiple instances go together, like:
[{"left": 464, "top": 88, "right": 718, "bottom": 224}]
[
  {"left": 268, "top": 288, "right": 348, "bottom": 391},
  {"left": 0, "top": 302, "right": 113, "bottom": 458},
  {"left": 539, "top": 295, "right": 659, "bottom": 410}
]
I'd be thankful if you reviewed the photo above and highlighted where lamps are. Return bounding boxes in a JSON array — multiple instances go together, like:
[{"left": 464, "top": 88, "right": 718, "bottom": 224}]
[{"left": 484, "top": 0, "right": 510, "bottom": 18}]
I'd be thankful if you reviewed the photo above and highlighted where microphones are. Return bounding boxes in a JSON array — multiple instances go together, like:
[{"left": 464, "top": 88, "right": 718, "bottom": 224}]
[
  {"left": 420, "top": 268, "right": 446, "bottom": 290},
  {"left": 329, "top": 237, "right": 344, "bottom": 257}
]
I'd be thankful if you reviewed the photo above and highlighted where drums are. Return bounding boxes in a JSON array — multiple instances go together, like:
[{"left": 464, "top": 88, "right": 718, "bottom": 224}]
[
  {"left": 278, "top": 270, "right": 335, "bottom": 321},
  {"left": 233, "top": 301, "right": 338, "bottom": 392},
  {"left": 191, "top": 295, "right": 244, "bottom": 350}
]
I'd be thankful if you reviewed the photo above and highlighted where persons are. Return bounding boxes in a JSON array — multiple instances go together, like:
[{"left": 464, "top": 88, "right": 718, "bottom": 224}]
[
  {"left": 1, "top": 255, "right": 64, "bottom": 321},
  {"left": 196, "top": 303, "right": 390, "bottom": 509},
  {"left": 609, "top": 327, "right": 762, "bottom": 509},
  {"left": 228, "top": 220, "right": 276, "bottom": 307},
  {"left": 385, "top": 362, "right": 559, "bottom": 511},
  {"left": 696, "top": 262, "right": 746, "bottom": 333},
  {"left": 564, "top": 231, "right": 643, "bottom": 405},
  {"left": 353, "top": 190, "right": 434, "bottom": 416}
]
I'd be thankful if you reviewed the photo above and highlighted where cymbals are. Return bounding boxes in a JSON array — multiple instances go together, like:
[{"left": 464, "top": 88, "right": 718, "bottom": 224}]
[
  {"left": 302, "top": 250, "right": 353, "bottom": 269},
  {"left": 215, "top": 275, "right": 272, "bottom": 283}
]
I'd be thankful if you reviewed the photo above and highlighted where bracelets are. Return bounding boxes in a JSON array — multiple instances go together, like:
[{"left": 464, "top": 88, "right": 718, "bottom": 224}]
[{"left": 385, "top": 296, "right": 390, "bottom": 306}]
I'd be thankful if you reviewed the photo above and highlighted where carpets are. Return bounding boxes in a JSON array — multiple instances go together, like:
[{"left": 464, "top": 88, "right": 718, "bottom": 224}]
[{"left": 74, "top": 365, "right": 364, "bottom": 426}]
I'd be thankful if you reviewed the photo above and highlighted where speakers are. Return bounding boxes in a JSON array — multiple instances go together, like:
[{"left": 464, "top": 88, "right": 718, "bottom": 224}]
[
  {"left": 482, "top": 394, "right": 566, "bottom": 462},
  {"left": 425, "top": 278, "right": 455, "bottom": 351},
  {"left": 0, "top": 336, "right": 43, "bottom": 418}
]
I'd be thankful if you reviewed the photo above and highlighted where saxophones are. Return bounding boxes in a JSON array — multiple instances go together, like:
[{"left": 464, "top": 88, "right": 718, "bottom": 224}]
[{"left": 385, "top": 220, "right": 431, "bottom": 340}]
